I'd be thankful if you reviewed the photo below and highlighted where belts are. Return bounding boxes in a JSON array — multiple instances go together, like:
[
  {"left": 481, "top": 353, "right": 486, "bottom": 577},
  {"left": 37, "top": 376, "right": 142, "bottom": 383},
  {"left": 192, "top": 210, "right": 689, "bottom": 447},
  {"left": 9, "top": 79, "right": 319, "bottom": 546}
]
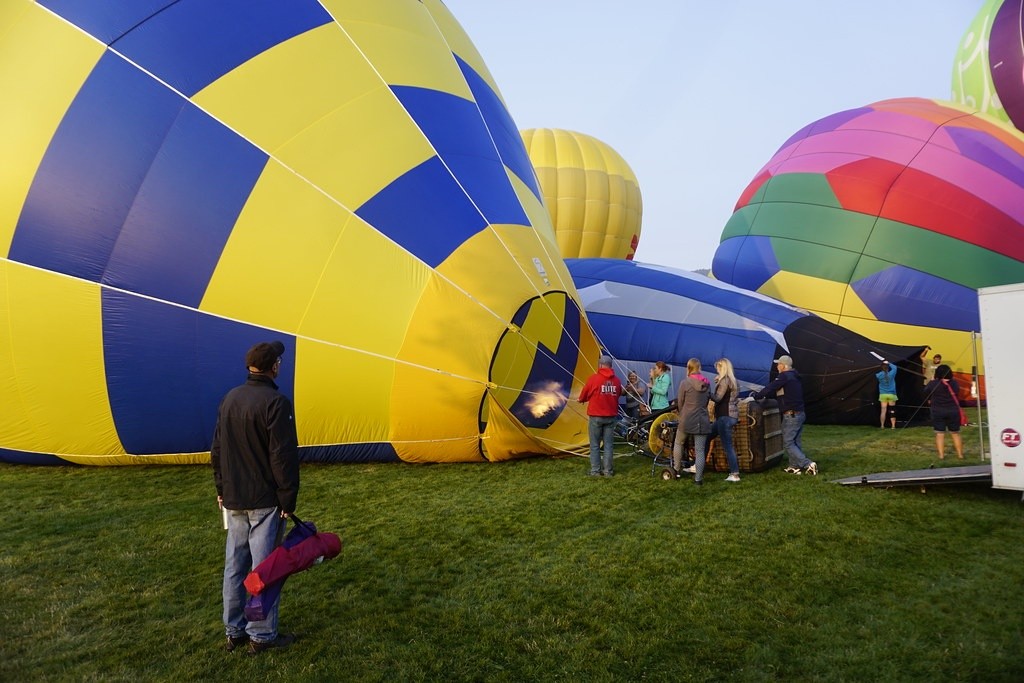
[{"left": 784, "top": 409, "right": 800, "bottom": 415}]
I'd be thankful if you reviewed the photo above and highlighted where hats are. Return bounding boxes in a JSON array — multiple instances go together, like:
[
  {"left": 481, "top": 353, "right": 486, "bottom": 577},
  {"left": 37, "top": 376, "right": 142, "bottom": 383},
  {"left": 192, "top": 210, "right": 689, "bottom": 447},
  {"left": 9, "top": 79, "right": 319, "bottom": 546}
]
[
  {"left": 774, "top": 355, "right": 793, "bottom": 365},
  {"left": 599, "top": 356, "right": 612, "bottom": 364},
  {"left": 246, "top": 340, "right": 285, "bottom": 373}
]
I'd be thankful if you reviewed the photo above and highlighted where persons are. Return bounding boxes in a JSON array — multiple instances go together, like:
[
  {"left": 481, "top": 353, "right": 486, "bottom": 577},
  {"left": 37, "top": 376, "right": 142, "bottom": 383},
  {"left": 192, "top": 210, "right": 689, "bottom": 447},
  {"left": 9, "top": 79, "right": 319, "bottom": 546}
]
[
  {"left": 924, "top": 366, "right": 965, "bottom": 459},
  {"left": 578, "top": 355, "right": 623, "bottom": 477},
  {"left": 673, "top": 358, "right": 712, "bottom": 484},
  {"left": 921, "top": 348, "right": 942, "bottom": 403},
  {"left": 749, "top": 356, "right": 819, "bottom": 475},
  {"left": 876, "top": 360, "right": 898, "bottom": 429},
  {"left": 209, "top": 340, "right": 300, "bottom": 654},
  {"left": 683, "top": 358, "right": 741, "bottom": 481},
  {"left": 620, "top": 361, "right": 672, "bottom": 420}
]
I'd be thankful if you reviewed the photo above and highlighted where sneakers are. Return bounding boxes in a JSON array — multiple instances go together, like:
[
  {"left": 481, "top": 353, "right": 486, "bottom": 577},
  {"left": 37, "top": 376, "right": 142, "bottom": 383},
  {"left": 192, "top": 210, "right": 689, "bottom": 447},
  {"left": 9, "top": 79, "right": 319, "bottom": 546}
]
[
  {"left": 227, "top": 636, "right": 250, "bottom": 652},
  {"left": 724, "top": 475, "right": 740, "bottom": 481},
  {"left": 249, "top": 632, "right": 295, "bottom": 655},
  {"left": 806, "top": 462, "right": 818, "bottom": 477},
  {"left": 683, "top": 464, "right": 696, "bottom": 473},
  {"left": 784, "top": 467, "right": 802, "bottom": 475}
]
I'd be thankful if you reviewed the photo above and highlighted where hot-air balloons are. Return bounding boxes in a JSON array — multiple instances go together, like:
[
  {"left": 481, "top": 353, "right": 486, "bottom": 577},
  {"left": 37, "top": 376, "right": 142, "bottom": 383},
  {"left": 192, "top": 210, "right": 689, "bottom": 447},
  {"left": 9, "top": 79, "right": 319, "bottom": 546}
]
[
  {"left": 518, "top": 127, "right": 643, "bottom": 262},
  {"left": 564, "top": 257, "right": 991, "bottom": 431},
  {"left": 1, "top": 0, "right": 790, "bottom": 478},
  {"left": 704, "top": 98, "right": 1024, "bottom": 405},
  {"left": 948, "top": 0, "right": 1024, "bottom": 134}
]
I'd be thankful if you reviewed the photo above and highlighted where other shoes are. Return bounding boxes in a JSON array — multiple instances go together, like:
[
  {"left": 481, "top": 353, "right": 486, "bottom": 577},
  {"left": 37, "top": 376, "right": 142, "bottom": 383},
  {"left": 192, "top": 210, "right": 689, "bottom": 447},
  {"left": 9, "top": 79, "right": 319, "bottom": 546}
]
[
  {"left": 586, "top": 472, "right": 600, "bottom": 476},
  {"left": 676, "top": 477, "right": 680, "bottom": 480},
  {"left": 694, "top": 480, "right": 703, "bottom": 487},
  {"left": 604, "top": 473, "right": 612, "bottom": 477}
]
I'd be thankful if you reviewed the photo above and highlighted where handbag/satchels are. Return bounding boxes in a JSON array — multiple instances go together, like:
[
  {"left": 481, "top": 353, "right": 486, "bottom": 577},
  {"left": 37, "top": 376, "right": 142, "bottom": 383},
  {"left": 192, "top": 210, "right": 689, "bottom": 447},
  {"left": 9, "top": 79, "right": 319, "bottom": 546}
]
[
  {"left": 960, "top": 410, "right": 969, "bottom": 426},
  {"left": 639, "top": 403, "right": 650, "bottom": 417}
]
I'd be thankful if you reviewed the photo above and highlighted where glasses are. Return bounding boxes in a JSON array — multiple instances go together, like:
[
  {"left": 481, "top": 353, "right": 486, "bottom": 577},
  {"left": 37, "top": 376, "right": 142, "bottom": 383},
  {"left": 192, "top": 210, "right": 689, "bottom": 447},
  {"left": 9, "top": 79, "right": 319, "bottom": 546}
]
[{"left": 277, "top": 355, "right": 282, "bottom": 364}]
